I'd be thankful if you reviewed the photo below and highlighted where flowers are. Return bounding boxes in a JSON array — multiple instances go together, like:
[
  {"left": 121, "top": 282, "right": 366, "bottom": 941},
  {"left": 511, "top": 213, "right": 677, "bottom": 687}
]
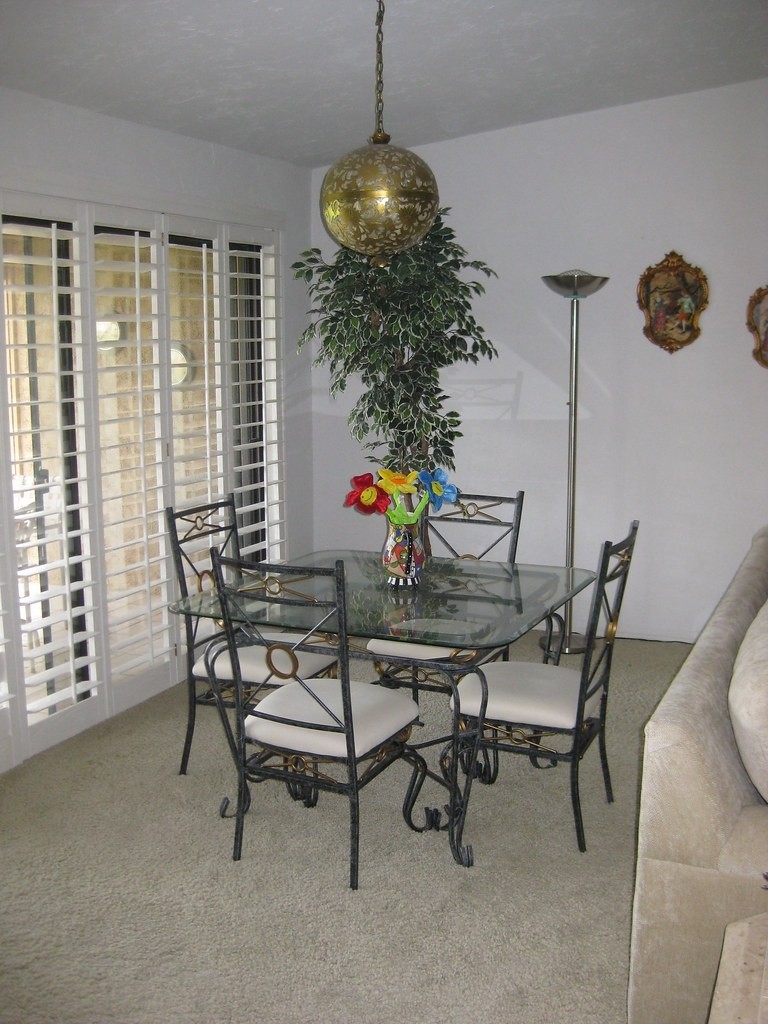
[{"left": 345, "top": 467, "right": 457, "bottom": 524}]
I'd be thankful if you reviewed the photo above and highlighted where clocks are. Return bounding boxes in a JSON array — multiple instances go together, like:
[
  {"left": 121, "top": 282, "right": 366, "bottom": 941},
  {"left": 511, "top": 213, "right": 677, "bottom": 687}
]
[{"left": 95, "top": 309, "right": 124, "bottom": 355}]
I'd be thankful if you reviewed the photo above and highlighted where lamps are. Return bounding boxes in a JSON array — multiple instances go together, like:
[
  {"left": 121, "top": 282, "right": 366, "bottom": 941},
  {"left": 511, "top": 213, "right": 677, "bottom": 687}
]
[
  {"left": 321, "top": 0, "right": 440, "bottom": 266},
  {"left": 538, "top": 269, "right": 610, "bottom": 654}
]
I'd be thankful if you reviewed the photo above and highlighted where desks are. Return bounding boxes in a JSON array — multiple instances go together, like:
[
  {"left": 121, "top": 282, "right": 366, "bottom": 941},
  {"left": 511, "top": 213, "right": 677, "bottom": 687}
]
[{"left": 166, "top": 549, "right": 597, "bottom": 867}]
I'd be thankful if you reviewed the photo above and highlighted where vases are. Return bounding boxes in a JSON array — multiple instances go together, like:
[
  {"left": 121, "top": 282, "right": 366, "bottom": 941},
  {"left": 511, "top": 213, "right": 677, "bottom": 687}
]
[{"left": 382, "top": 511, "right": 426, "bottom": 578}]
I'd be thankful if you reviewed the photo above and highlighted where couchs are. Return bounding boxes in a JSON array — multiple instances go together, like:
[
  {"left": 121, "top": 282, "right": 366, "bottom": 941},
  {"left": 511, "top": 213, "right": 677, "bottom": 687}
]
[{"left": 627, "top": 523, "right": 768, "bottom": 1024}]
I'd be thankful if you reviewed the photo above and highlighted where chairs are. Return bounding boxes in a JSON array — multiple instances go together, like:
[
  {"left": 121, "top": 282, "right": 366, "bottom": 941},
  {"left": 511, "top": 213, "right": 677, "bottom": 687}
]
[
  {"left": 210, "top": 547, "right": 432, "bottom": 890},
  {"left": 165, "top": 493, "right": 350, "bottom": 775},
  {"left": 366, "top": 491, "right": 523, "bottom": 696},
  {"left": 448, "top": 520, "right": 640, "bottom": 852}
]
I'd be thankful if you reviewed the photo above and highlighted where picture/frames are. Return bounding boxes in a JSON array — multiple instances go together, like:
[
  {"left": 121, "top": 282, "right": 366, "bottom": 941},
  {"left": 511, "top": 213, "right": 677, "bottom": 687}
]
[
  {"left": 636, "top": 250, "right": 709, "bottom": 355},
  {"left": 745, "top": 284, "right": 768, "bottom": 369}
]
